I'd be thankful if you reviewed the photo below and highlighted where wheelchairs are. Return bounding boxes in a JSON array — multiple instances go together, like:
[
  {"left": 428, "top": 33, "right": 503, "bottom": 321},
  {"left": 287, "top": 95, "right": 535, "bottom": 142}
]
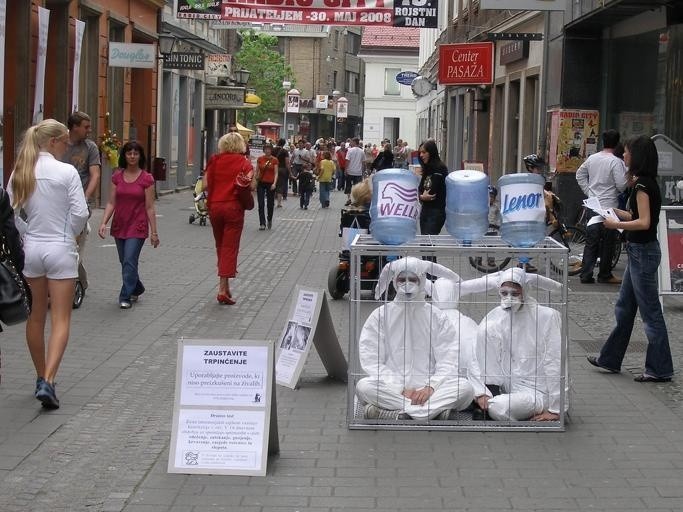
[{"left": 327, "top": 208, "right": 402, "bottom": 303}]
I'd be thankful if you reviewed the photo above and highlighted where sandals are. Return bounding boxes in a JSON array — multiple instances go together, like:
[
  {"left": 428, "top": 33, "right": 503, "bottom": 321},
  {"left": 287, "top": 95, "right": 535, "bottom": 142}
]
[
  {"left": 634, "top": 374, "right": 673, "bottom": 382},
  {"left": 587, "top": 356, "right": 618, "bottom": 374}
]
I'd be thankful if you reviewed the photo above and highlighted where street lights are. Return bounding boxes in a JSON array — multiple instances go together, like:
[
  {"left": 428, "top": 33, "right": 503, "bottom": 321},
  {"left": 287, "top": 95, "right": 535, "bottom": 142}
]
[
  {"left": 333, "top": 90, "right": 341, "bottom": 138},
  {"left": 282, "top": 80, "right": 292, "bottom": 140},
  {"left": 243, "top": 88, "right": 256, "bottom": 127}
]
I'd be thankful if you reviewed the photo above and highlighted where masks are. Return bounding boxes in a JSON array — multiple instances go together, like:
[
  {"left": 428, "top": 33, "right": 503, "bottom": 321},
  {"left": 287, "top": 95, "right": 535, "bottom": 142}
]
[
  {"left": 397, "top": 283, "right": 418, "bottom": 299},
  {"left": 501, "top": 296, "right": 522, "bottom": 311}
]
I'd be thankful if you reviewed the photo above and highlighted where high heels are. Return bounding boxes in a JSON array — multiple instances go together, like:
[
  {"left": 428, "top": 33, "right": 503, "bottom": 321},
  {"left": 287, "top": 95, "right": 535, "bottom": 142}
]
[
  {"left": 225, "top": 290, "right": 232, "bottom": 297},
  {"left": 277, "top": 205, "right": 283, "bottom": 208},
  {"left": 217, "top": 295, "right": 236, "bottom": 305}
]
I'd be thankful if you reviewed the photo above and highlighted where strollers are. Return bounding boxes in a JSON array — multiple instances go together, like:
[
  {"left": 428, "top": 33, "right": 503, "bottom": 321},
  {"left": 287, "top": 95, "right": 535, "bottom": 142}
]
[
  {"left": 188, "top": 176, "right": 208, "bottom": 226},
  {"left": 72, "top": 200, "right": 92, "bottom": 309}
]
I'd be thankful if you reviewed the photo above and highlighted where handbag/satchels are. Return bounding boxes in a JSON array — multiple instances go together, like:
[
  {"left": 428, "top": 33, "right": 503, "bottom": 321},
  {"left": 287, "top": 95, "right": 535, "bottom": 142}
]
[
  {"left": 0, "top": 232, "right": 32, "bottom": 325},
  {"left": 256, "top": 155, "right": 272, "bottom": 184},
  {"left": 234, "top": 156, "right": 255, "bottom": 210}
]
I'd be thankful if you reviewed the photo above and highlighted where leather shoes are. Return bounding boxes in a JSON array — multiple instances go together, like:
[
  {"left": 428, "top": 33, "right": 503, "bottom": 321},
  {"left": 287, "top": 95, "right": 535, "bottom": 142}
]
[
  {"left": 132, "top": 292, "right": 139, "bottom": 301},
  {"left": 322, "top": 204, "right": 325, "bottom": 208},
  {"left": 37, "top": 383, "right": 59, "bottom": 408},
  {"left": 325, "top": 201, "right": 329, "bottom": 207},
  {"left": 35, "top": 378, "right": 44, "bottom": 394},
  {"left": 267, "top": 221, "right": 272, "bottom": 229},
  {"left": 120, "top": 300, "right": 131, "bottom": 308},
  {"left": 259, "top": 225, "right": 265, "bottom": 230}
]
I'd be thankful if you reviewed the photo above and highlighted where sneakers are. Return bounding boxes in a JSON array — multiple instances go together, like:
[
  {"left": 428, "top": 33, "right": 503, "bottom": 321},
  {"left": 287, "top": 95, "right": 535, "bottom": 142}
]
[
  {"left": 597, "top": 274, "right": 622, "bottom": 283},
  {"left": 582, "top": 277, "right": 596, "bottom": 284},
  {"left": 437, "top": 409, "right": 450, "bottom": 419},
  {"left": 300, "top": 204, "right": 303, "bottom": 208},
  {"left": 304, "top": 207, "right": 307, "bottom": 210},
  {"left": 363, "top": 403, "right": 402, "bottom": 420},
  {"left": 519, "top": 263, "right": 536, "bottom": 271}
]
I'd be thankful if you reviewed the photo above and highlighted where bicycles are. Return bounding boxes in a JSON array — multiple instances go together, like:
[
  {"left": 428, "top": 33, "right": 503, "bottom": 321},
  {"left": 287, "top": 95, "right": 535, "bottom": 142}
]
[{"left": 468, "top": 190, "right": 586, "bottom": 276}]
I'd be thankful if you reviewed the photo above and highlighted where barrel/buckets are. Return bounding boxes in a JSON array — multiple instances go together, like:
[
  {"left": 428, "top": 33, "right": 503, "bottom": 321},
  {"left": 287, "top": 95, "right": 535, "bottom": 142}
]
[
  {"left": 370, "top": 168, "right": 420, "bottom": 262},
  {"left": 445, "top": 170, "right": 490, "bottom": 249},
  {"left": 498, "top": 173, "right": 546, "bottom": 263}
]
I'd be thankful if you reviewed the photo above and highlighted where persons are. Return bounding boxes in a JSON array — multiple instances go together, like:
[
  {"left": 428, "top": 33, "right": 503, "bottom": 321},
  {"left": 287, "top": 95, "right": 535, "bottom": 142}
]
[
  {"left": 576, "top": 129, "right": 628, "bottom": 284},
  {"left": 255, "top": 144, "right": 279, "bottom": 230},
  {"left": 0, "top": 187, "right": 32, "bottom": 332},
  {"left": 99, "top": 141, "right": 160, "bottom": 308},
  {"left": 587, "top": 136, "right": 674, "bottom": 382},
  {"left": 475, "top": 186, "right": 503, "bottom": 268},
  {"left": 418, "top": 141, "right": 449, "bottom": 283},
  {"left": 63, "top": 111, "right": 102, "bottom": 203},
  {"left": 278, "top": 137, "right": 365, "bottom": 211},
  {"left": 356, "top": 258, "right": 473, "bottom": 421},
  {"left": 5, "top": 118, "right": 90, "bottom": 409},
  {"left": 516, "top": 155, "right": 556, "bottom": 273},
  {"left": 352, "top": 151, "right": 398, "bottom": 299},
  {"left": 203, "top": 131, "right": 255, "bottom": 304},
  {"left": 431, "top": 278, "right": 480, "bottom": 380},
  {"left": 466, "top": 268, "right": 571, "bottom": 422},
  {"left": 367, "top": 137, "right": 411, "bottom": 170}
]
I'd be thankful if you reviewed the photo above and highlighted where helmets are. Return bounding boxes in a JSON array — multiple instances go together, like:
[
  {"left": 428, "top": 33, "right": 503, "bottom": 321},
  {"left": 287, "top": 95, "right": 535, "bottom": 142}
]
[{"left": 524, "top": 154, "right": 544, "bottom": 167}]
